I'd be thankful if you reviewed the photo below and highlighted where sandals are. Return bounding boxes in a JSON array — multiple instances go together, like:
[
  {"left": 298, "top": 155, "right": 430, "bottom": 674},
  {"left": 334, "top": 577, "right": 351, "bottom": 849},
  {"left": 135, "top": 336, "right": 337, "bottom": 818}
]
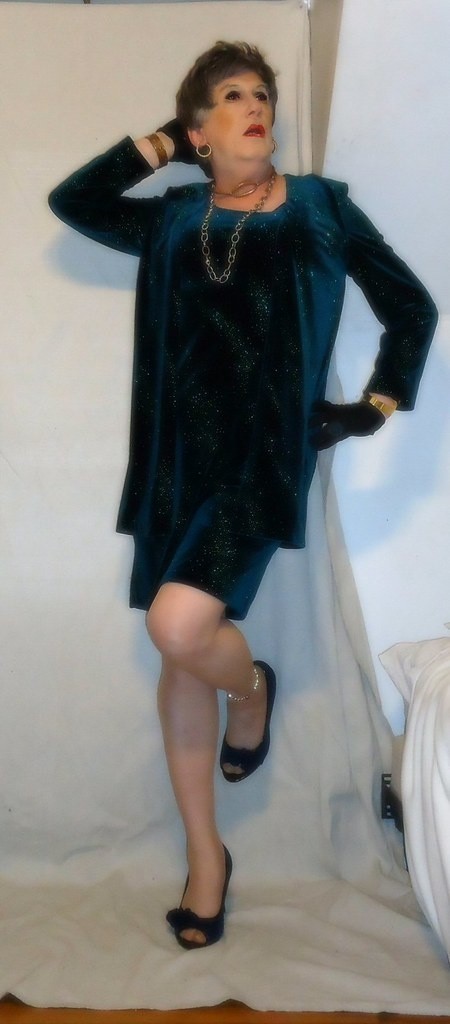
[
  {"left": 220, "top": 660, "right": 276, "bottom": 782},
  {"left": 167, "top": 843, "right": 233, "bottom": 949}
]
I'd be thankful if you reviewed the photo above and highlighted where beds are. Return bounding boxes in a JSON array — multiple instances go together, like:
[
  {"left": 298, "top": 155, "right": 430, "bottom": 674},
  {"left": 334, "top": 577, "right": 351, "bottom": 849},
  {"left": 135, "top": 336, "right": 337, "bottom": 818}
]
[{"left": 369, "top": 637, "right": 450, "bottom": 952}]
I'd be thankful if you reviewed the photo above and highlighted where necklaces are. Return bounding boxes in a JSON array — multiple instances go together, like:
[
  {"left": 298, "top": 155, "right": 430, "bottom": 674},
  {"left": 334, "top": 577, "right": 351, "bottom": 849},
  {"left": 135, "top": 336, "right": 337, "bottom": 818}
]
[{"left": 201, "top": 168, "right": 278, "bottom": 284}]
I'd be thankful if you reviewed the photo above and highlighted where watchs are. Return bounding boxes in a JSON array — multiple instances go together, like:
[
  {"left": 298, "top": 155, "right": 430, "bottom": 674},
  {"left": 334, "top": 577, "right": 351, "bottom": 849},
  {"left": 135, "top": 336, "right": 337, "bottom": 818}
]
[{"left": 363, "top": 394, "right": 395, "bottom": 418}]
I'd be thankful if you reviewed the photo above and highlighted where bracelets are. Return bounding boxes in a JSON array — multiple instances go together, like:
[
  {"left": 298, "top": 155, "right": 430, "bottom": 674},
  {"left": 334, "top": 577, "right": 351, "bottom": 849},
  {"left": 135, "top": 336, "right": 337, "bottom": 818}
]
[{"left": 144, "top": 134, "right": 168, "bottom": 169}]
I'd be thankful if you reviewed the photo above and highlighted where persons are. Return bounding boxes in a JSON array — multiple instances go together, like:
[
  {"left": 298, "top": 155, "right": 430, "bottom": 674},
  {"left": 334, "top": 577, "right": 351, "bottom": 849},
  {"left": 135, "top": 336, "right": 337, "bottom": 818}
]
[{"left": 47, "top": 41, "right": 437, "bottom": 949}]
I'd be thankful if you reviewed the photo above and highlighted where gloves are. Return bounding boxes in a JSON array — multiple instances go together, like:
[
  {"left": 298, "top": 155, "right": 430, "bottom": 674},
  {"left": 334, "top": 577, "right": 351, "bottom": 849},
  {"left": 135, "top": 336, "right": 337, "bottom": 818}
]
[
  {"left": 158, "top": 119, "right": 215, "bottom": 179},
  {"left": 311, "top": 400, "right": 386, "bottom": 451}
]
